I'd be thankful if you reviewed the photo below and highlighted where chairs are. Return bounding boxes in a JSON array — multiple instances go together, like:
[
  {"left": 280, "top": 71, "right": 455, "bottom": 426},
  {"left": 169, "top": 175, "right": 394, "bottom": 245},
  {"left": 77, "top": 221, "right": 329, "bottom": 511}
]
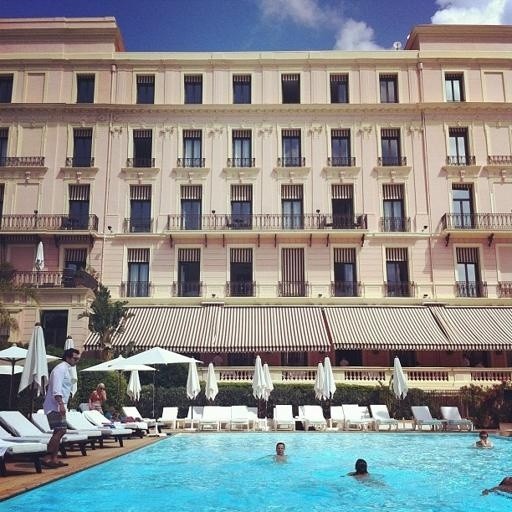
[
  {"left": 156, "top": 404, "right": 477, "bottom": 432},
  {"left": 1, "top": 405, "right": 160, "bottom": 477}
]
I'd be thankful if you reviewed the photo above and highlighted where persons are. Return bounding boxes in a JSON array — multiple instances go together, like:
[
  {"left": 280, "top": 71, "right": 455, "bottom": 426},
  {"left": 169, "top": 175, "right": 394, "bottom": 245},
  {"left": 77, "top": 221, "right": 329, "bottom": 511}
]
[
  {"left": 87, "top": 381, "right": 107, "bottom": 417},
  {"left": 483, "top": 475, "right": 512, "bottom": 498},
  {"left": 39, "top": 347, "right": 81, "bottom": 469},
  {"left": 473, "top": 429, "right": 494, "bottom": 451},
  {"left": 273, "top": 441, "right": 288, "bottom": 463},
  {"left": 346, "top": 459, "right": 370, "bottom": 477}
]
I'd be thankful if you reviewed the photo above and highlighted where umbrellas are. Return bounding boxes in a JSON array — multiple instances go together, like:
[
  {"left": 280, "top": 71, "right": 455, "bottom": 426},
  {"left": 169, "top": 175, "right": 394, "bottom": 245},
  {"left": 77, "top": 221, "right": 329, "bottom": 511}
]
[{"left": 31, "top": 237, "right": 45, "bottom": 281}]
